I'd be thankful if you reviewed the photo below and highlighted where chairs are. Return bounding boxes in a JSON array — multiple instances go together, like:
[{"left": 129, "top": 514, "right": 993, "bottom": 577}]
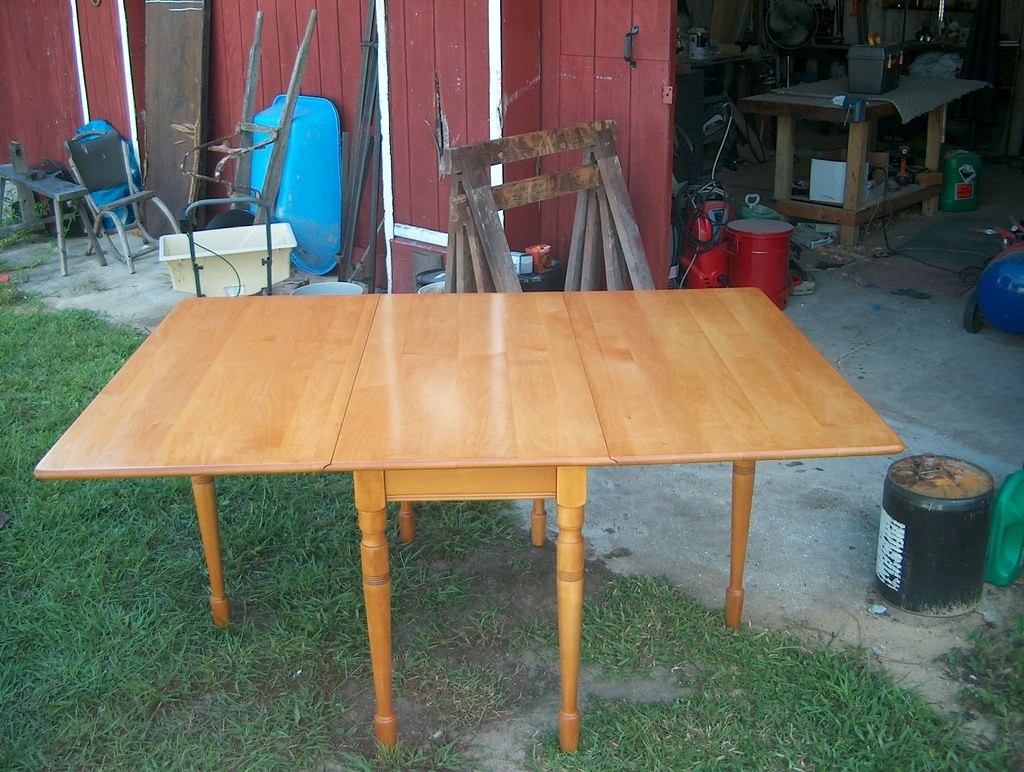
[{"left": 64, "top": 129, "right": 183, "bottom": 275}]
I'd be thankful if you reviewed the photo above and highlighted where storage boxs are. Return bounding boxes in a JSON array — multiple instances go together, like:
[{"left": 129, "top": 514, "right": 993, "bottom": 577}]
[
  {"left": 809, "top": 147, "right": 890, "bottom": 233},
  {"left": 159, "top": 221, "right": 299, "bottom": 297}
]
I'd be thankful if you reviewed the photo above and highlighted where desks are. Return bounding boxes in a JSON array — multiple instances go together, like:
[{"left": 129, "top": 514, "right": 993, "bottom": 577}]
[
  {"left": 682, "top": 49, "right": 776, "bottom": 161},
  {"left": 737, "top": 75, "right": 993, "bottom": 243},
  {"left": 34, "top": 287, "right": 904, "bottom": 754}
]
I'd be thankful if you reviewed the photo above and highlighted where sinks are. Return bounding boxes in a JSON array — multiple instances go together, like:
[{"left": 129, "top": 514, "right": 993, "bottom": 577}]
[{"left": 157, "top": 222, "right": 298, "bottom": 298}]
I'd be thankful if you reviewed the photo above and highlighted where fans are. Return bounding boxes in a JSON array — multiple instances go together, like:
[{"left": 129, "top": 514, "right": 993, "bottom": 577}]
[{"left": 766, "top": 0, "right": 819, "bottom": 87}]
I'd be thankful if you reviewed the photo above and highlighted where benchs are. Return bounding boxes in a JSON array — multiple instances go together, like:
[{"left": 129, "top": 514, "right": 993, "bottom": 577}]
[{"left": 0, "top": 163, "right": 108, "bottom": 277}]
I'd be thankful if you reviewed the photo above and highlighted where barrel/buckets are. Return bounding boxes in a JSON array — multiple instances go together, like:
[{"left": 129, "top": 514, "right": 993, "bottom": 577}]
[
  {"left": 413, "top": 268, "right": 448, "bottom": 294},
  {"left": 875, "top": 455, "right": 997, "bottom": 618},
  {"left": 941, "top": 149, "right": 982, "bottom": 211},
  {"left": 289, "top": 281, "right": 364, "bottom": 296}
]
